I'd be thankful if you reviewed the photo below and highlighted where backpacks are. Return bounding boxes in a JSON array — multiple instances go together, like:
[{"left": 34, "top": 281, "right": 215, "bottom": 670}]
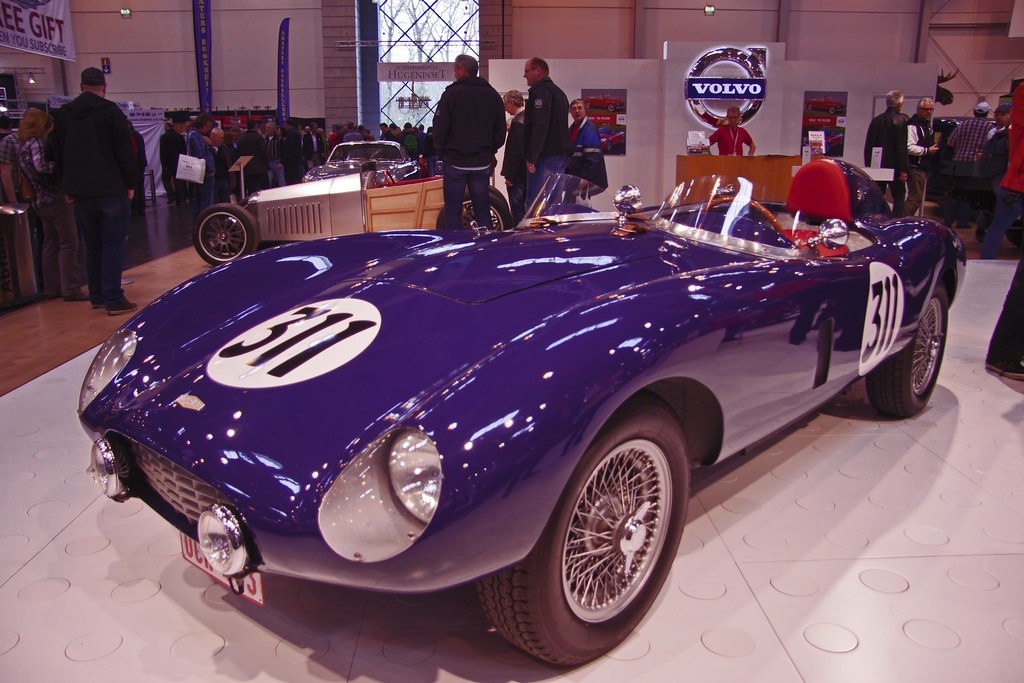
[{"left": 403, "top": 127, "right": 418, "bottom": 155}]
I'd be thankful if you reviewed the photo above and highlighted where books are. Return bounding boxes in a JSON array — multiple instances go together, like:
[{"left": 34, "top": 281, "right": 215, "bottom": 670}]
[
  {"left": 807, "top": 131, "right": 826, "bottom": 154},
  {"left": 686, "top": 131, "right": 710, "bottom": 154}
]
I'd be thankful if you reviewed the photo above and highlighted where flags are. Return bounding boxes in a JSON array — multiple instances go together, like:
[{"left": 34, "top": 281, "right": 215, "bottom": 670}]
[{"left": 558, "top": 120, "right": 608, "bottom": 197}]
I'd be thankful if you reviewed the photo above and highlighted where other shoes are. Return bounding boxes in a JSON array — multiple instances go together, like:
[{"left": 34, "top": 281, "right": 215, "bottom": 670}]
[
  {"left": 64, "top": 293, "right": 89, "bottom": 301},
  {"left": 167, "top": 196, "right": 177, "bottom": 204},
  {"left": 956, "top": 222, "right": 971, "bottom": 229},
  {"left": 96, "top": 296, "right": 138, "bottom": 316},
  {"left": 985, "top": 360, "right": 1024, "bottom": 380},
  {"left": 975, "top": 234, "right": 984, "bottom": 241},
  {"left": 46, "top": 291, "right": 61, "bottom": 299}
]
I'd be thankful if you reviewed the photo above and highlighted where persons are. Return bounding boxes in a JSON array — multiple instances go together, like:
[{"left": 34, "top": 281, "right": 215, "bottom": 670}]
[
  {"left": 433, "top": 54, "right": 505, "bottom": 230},
  {"left": 0, "top": 108, "right": 89, "bottom": 302},
  {"left": 560, "top": 371, "right": 622, "bottom": 457},
  {"left": 569, "top": 99, "right": 608, "bottom": 186},
  {"left": 51, "top": 67, "right": 138, "bottom": 315},
  {"left": 500, "top": 449, "right": 542, "bottom": 499},
  {"left": 522, "top": 57, "right": 569, "bottom": 219},
  {"left": 126, "top": 121, "right": 146, "bottom": 202},
  {"left": 864, "top": 81, "right": 1024, "bottom": 381},
  {"left": 501, "top": 90, "right": 526, "bottom": 228},
  {"left": 159, "top": 121, "right": 187, "bottom": 204},
  {"left": 189, "top": 113, "right": 433, "bottom": 224},
  {"left": 787, "top": 277, "right": 865, "bottom": 351},
  {"left": 703, "top": 307, "right": 752, "bottom": 341},
  {"left": 708, "top": 106, "right": 756, "bottom": 156}
]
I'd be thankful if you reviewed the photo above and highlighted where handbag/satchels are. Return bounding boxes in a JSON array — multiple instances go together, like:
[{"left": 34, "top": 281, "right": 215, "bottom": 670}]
[
  {"left": 175, "top": 130, "right": 206, "bottom": 184},
  {"left": 17, "top": 168, "right": 35, "bottom": 201},
  {"left": 988, "top": 127, "right": 1009, "bottom": 174},
  {"left": 244, "top": 148, "right": 268, "bottom": 177}
]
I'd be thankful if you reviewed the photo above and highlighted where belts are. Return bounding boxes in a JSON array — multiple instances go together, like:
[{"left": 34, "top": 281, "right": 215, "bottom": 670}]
[{"left": 313, "top": 151, "right": 319, "bottom": 153}]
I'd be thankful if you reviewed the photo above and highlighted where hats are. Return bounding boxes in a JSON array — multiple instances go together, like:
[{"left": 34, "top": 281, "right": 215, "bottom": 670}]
[
  {"left": 994, "top": 102, "right": 1012, "bottom": 112},
  {"left": 82, "top": 67, "right": 107, "bottom": 87},
  {"left": 347, "top": 122, "right": 354, "bottom": 127},
  {"left": 975, "top": 102, "right": 991, "bottom": 114}
]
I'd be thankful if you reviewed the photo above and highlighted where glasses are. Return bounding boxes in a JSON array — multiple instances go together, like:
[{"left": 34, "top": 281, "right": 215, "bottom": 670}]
[{"left": 921, "top": 107, "right": 934, "bottom": 112}]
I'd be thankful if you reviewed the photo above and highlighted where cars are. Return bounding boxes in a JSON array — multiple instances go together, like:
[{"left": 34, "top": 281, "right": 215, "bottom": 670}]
[
  {"left": 803, "top": 128, "right": 844, "bottom": 153},
  {"left": 597, "top": 126, "right": 624, "bottom": 152},
  {"left": 192, "top": 160, "right": 515, "bottom": 267},
  {"left": 301, "top": 141, "right": 422, "bottom": 189}
]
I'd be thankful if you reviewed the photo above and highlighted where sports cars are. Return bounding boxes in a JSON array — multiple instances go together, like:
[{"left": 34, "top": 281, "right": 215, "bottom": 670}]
[
  {"left": 585, "top": 92, "right": 625, "bottom": 112},
  {"left": 804, "top": 95, "right": 845, "bottom": 114},
  {"left": 78, "top": 158, "right": 971, "bottom": 667}
]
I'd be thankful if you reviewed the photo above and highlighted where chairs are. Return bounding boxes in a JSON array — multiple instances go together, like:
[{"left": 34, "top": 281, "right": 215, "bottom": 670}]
[{"left": 783, "top": 160, "right": 850, "bottom": 258}]
